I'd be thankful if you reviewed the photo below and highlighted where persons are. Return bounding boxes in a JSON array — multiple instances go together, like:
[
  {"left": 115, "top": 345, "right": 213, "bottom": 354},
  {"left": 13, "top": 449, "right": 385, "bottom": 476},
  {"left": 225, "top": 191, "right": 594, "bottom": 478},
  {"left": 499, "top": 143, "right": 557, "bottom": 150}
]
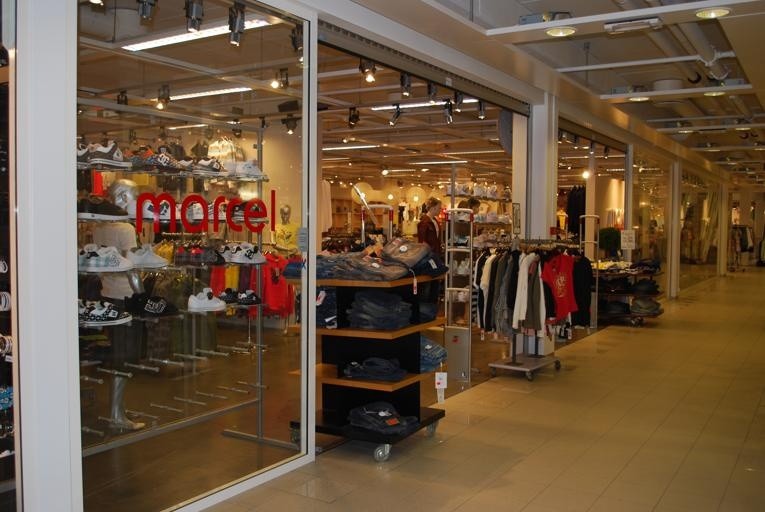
[
  {"left": 93, "top": 179, "right": 147, "bottom": 431},
  {"left": 632, "top": 219, "right": 662, "bottom": 265},
  {"left": 416, "top": 196, "right": 443, "bottom": 304},
  {"left": 269, "top": 203, "right": 299, "bottom": 252},
  {"left": 456, "top": 200, "right": 470, "bottom": 214},
  {"left": 468, "top": 197, "right": 480, "bottom": 214}
]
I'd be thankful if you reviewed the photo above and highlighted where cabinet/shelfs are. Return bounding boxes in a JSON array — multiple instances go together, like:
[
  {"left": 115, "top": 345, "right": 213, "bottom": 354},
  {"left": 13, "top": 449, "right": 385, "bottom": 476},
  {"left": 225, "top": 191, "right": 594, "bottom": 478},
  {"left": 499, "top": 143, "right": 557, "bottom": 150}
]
[{"left": 0, "top": 96, "right": 665, "bottom": 498}]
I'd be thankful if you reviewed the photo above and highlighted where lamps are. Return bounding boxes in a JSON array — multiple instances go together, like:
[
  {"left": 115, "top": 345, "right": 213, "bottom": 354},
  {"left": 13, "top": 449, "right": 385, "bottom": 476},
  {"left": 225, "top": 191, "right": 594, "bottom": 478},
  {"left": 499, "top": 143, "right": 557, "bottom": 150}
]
[
  {"left": 182, "top": 2, "right": 205, "bottom": 34},
  {"left": 425, "top": 83, "right": 439, "bottom": 95},
  {"left": 476, "top": 99, "right": 487, "bottom": 120},
  {"left": 274, "top": 69, "right": 290, "bottom": 90},
  {"left": 348, "top": 107, "right": 362, "bottom": 129},
  {"left": 232, "top": 107, "right": 244, "bottom": 139},
  {"left": 388, "top": 103, "right": 407, "bottom": 128},
  {"left": 155, "top": 84, "right": 172, "bottom": 112},
  {"left": 399, "top": 72, "right": 411, "bottom": 97},
  {"left": 441, "top": 98, "right": 456, "bottom": 124},
  {"left": 357, "top": 58, "right": 376, "bottom": 84},
  {"left": 454, "top": 90, "right": 464, "bottom": 114},
  {"left": 276, "top": 101, "right": 300, "bottom": 136},
  {"left": 135, "top": 1, "right": 158, "bottom": 20},
  {"left": 289, "top": 23, "right": 304, "bottom": 69},
  {"left": 519, "top": 5, "right": 764, "bottom": 159},
  {"left": 228, "top": 1, "right": 247, "bottom": 47}
]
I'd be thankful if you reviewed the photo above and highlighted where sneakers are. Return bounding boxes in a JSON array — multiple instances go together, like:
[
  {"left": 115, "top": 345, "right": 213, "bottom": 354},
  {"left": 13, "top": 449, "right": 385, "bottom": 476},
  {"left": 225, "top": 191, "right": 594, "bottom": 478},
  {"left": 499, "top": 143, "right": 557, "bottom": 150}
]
[
  {"left": 78, "top": 196, "right": 270, "bottom": 223},
  {"left": 78, "top": 239, "right": 267, "bottom": 274},
  {"left": 448, "top": 212, "right": 512, "bottom": 225},
  {"left": 448, "top": 232, "right": 512, "bottom": 249},
  {"left": 446, "top": 184, "right": 512, "bottom": 200},
  {"left": 78, "top": 292, "right": 179, "bottom": 326},
  {"left": 77, "top": 142, "right": 268, "bottom": 181},
  {"left": 187, "top": 287, "right": 261, "bottom": 311},
  {"left": 453, "top": 260, "right": 470, "bottom": 275},
  {"left": 458, "top": 292, "right": 469, "bottom": 301}
]
